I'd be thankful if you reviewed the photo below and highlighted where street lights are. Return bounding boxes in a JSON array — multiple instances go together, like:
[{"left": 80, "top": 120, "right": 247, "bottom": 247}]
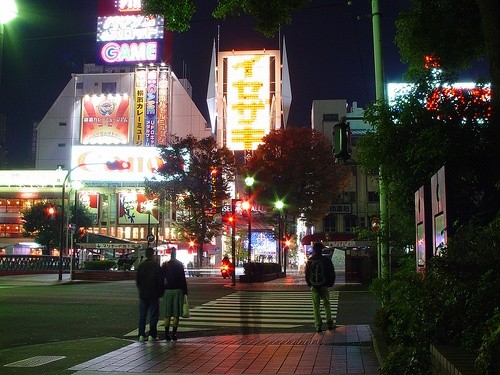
[
  {"left": 115, "top": 190, "right": 135, "bottom": 240},
  {"left": 73, "top": 181, "right": 81, "bottom": 235},
  {"left": 229, "top": 199, "right": 251, "bottom": 285},
  {"left": 244, "top": 168, "right": 254, "bottom": 281},
  {"left": 275, "top": 197, "right": 284, "bottom": 278},
  {"left": 282, "top": 234, "right": 295, "bottom": 277},
  {"left": 142, "top": 201, "right": 155, "bottom": 239}
]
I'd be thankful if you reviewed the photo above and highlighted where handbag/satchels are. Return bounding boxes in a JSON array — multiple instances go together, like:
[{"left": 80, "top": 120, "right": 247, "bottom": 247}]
[{"left": 182, "top": 294, "right": 190, "bottom": 318}]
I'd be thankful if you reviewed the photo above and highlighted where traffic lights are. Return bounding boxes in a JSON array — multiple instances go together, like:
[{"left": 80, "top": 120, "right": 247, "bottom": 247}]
[{"left": 107, "top": 161, "right": 132, "bottom": 171}]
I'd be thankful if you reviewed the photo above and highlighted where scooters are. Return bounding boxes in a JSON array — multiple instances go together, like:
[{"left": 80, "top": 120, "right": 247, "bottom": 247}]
[{"left": 221, "top": 263, "right": 233, "bottom": 279}]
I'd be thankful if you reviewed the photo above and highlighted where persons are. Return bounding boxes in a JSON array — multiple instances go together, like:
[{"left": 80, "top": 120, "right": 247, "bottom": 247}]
[
  {"left": 221, "top": 254, "right": 234, "bottom": 279},
  {"left": 0, "top": 246, "right": 101, "bottom": 270},
  {"left": 305, "top": 243, "right": 337, "bottom": 334},
  {"left": 124, "top": 254, "right": 131, "bottom": 271},
  {"left": 135, "top": 247, "right": 165, "bottom": 342},
  {"left": 162, "top": 247, "right": 188, "bottom": 341},
  {"left": 117, "top": 255, "right": 124, "bottom": 269}
]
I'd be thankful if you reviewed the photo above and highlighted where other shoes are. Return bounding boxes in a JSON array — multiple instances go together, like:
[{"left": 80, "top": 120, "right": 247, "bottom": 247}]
[
  {"left": 315, "top": 326, "right": 322, "bottom": 332},
  {"left": 139, "top": 336, "right": 144, "bottom": 341},
  {"left": 165, "top": 334, "right": 172, "bottom": 341},
  {"left": 171, "top": 333, "right": 177, "bottom": 341},
  {"left": 148, "top": 335, "right": 159, "bottom": 341},
  {"left": 328, "top": 324, "right": 336, "bottom": 330}
]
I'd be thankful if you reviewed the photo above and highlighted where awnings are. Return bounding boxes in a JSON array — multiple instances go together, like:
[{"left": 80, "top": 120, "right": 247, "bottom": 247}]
[{"left": 301, "top": 231, "right": 378, "bottom": 249}]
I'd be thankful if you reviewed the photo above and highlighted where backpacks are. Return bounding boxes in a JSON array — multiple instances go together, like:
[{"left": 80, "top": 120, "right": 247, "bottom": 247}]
[{"left": 309, "top": 258, "right": 329, "bottom": 286}]
